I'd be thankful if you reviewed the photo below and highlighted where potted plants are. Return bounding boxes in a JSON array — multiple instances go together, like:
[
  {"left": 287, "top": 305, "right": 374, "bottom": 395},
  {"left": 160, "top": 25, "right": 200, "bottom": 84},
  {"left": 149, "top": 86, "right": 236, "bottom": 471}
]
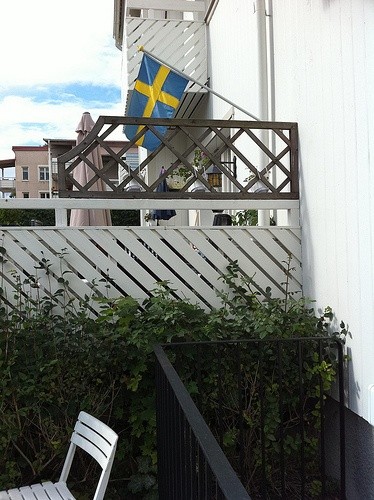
[
  {"left": 242, "top": 165, "right": 270, "bottom": 194},
  {"left": 119, "top": 165, "right": 147, "bottom": 192},
  {"left": 162, "top": 149, "right": 213, "bottom": 192}
]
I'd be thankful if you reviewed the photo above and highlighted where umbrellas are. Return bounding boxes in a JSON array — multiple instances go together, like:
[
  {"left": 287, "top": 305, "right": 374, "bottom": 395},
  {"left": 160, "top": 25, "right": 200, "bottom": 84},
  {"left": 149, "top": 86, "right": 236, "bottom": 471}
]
[
  {"left": 149, "top": 165, "right": 176, "bottom": 220},
  {"left": 70, "top": 112, "right": 112, "bottom": 227}
]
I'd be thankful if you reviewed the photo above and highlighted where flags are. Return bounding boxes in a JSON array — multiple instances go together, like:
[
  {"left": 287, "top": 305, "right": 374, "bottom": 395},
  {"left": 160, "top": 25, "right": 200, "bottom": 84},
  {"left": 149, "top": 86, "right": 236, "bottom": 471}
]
[{"left": 122, "top": 54, "right": 189, "bottom": 153}]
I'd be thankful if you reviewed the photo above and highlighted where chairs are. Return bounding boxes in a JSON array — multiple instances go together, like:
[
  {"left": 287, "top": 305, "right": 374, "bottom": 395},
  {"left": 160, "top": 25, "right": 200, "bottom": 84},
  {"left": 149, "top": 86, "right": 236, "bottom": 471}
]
[{"left": 0, "top": 411, "right": 120, "bottom": 500}]
